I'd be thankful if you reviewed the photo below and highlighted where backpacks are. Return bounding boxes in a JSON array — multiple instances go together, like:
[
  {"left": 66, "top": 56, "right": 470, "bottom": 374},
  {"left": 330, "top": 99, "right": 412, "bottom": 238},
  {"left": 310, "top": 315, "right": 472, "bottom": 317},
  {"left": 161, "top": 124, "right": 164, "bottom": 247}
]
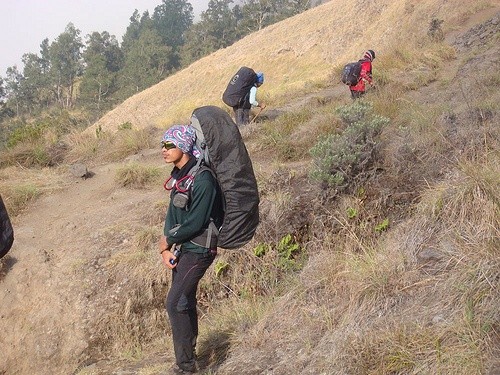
[
  {"left": 222, "top": 66, "right": 257, "bottom": 107},
  {"left": 188, "top": 105, "right": 260, "bottom": 249},
  {"left": 0, "top": 195, "right": 14, "bottom": 259},
  {"left": 342, "top": 62, "right": 362, "bottom": 86}
]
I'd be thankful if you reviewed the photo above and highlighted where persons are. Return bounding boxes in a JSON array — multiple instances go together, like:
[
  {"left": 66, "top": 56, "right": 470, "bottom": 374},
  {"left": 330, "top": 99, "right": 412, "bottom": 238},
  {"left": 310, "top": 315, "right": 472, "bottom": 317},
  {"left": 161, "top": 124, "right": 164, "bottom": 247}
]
[
  {"left": 346, "top": 47, "right": 380, "bottom": 103},
  {"left": 228, "top": 65, "right": 265, "bottom": 124},
  {"left": 155, "top": 119, "right": 221, "bottom": 374}
]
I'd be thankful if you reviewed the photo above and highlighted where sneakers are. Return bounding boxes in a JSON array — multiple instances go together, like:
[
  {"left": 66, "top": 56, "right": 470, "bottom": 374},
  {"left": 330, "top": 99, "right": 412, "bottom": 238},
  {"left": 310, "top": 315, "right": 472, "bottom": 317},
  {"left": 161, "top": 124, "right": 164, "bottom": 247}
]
[{"left": 167, "top": 364, "right": 194, "bottom": 375}]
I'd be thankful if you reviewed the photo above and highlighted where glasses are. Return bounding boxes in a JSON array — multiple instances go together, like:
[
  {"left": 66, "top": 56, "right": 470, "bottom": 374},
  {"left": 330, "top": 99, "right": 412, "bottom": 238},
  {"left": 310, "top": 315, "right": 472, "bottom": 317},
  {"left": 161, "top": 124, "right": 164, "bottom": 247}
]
[{"left": 161, "top": 141, "right": 176, "bottom": 150}]
